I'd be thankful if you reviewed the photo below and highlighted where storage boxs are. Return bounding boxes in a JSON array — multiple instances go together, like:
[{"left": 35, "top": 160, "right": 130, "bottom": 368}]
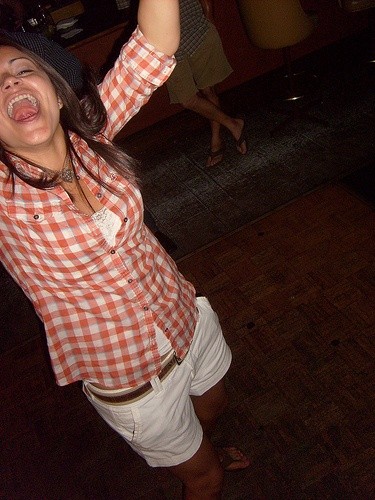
[{"left": 49, "top": 0, "right": 86, "bottom": 23}]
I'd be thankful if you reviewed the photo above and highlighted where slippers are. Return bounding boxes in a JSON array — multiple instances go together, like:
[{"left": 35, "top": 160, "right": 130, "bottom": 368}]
[{"left": 215, "top": 447, "right": 250, "bottom": 472}]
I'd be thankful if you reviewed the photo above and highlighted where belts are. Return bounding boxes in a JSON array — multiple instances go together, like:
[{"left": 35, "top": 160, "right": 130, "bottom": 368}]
[{"left": 85, "top": 351, "right": 182, "bottom": 407}]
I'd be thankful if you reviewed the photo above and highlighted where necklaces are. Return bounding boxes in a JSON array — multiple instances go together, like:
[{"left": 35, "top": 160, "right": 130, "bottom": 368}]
[{"left": 41, "top": 159, "right": 76, "bottom": 184}]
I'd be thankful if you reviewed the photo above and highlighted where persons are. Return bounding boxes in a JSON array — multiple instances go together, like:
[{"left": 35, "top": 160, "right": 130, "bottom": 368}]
[
  {"left": 164, "top": 0, "right": 248, "bottom": 168},
  {"left": 0, "top": 0, "right": 250, "bottom": 500}
]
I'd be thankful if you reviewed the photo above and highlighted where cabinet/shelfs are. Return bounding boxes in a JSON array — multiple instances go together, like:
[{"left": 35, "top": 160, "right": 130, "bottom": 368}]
[{"left": 62, "top": 0, "right": 375, "bottom": 142}]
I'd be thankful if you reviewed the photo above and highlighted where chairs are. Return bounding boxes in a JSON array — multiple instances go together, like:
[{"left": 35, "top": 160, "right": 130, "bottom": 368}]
[{"left": 235, "top": 0, "right": 320, "bottom": 103}]
